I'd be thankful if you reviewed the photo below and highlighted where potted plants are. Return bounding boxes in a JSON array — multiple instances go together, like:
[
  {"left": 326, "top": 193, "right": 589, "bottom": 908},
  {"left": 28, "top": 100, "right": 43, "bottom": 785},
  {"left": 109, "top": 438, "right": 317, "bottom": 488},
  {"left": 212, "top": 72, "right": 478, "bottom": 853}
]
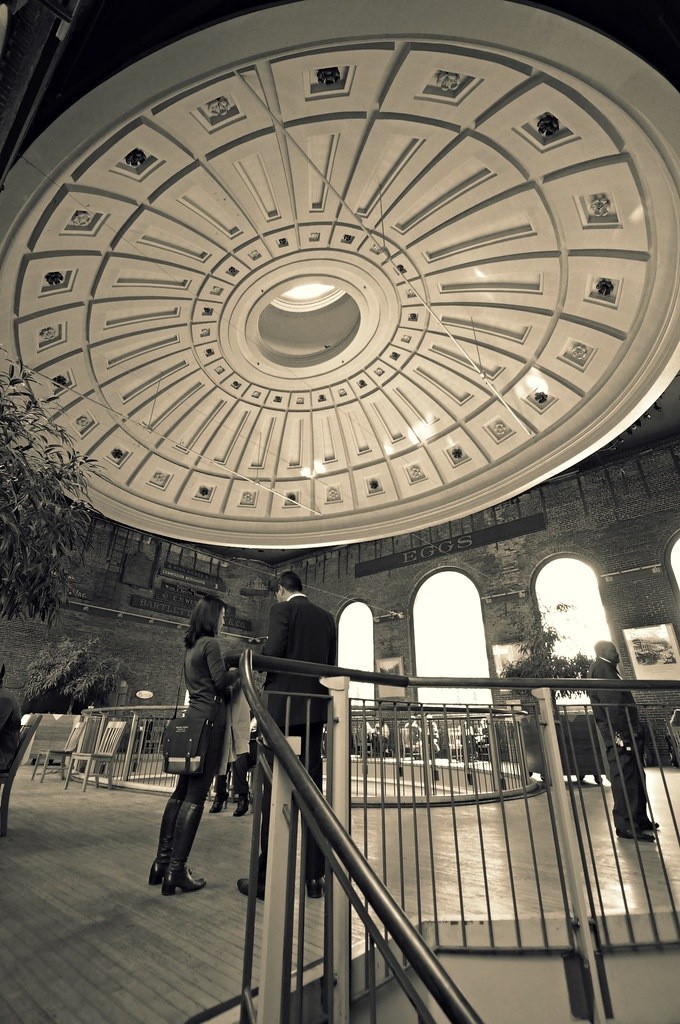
[
  {"left": 498, "top": 599, "right": 607, "bottom": 784},
  {"left": 23, "top": 634, "right": 120, "bottom": 764}
]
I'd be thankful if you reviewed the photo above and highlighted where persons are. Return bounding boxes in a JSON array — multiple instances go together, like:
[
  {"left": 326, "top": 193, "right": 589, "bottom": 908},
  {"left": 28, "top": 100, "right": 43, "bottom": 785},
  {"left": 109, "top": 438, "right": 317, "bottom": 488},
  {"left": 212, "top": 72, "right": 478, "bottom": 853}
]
[
  {"left": 238, "top": 571, "right": 338, "bottom": 898},
  {"left": 587, "top": 641, "right": 660, "bottom": 842},
  {"left": 210, "top": 666, "right": 251, "bottom": 817},
  {"left": 0, "top": 661, "right": 21, "bottom": 772},
  {"left": 148, "top": 595, "right": 241, "bottom": 897}
]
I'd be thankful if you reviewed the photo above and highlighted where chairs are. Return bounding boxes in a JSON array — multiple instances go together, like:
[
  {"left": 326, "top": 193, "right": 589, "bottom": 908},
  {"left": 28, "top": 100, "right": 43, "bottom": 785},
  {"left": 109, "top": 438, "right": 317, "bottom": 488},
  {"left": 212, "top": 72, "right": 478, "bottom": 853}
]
[
  {"left": 0, "top": 714, "right": 43, "bottom": 837},
  {"left": 64, "top": 721, "right": 127, "bottom": 793},
  {"left": 31, "top": 722, "right": 87, "bottom": 784}
]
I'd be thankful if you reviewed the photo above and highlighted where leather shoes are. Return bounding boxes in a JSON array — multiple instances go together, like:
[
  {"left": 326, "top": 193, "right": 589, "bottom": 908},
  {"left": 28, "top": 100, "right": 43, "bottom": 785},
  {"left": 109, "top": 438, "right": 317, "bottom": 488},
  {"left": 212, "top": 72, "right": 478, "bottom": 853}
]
[
  {"left": 237, "top": 878, "right": 266, "bottom": 901},
  {"left": 616, "top": 829, "right": 655, "bottom": 842},
  {"left": 635, "top": 821, "right": 659, "bottom": 830},
  {"left": 233, "top": 792, "right": 249, "bottom": 816},
  {"left": 209, "top": 792, "right": 230, "bottom": 813},
  {"left": 306, "top": 881, "right": 326, "bottom": 898}
]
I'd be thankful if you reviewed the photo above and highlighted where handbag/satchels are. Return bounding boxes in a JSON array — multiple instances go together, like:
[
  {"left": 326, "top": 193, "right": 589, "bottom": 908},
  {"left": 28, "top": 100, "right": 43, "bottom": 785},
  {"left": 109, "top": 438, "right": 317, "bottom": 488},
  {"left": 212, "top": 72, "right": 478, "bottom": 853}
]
[{"left": 161, "top": 717, "right": 213, "bottom": 776}]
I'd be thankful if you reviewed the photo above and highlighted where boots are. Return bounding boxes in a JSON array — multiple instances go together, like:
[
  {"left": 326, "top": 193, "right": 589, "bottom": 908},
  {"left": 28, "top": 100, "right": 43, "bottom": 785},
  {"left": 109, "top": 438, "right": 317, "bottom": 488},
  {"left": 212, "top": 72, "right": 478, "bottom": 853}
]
[
  {"left": 161, "top": 801, "right": 207, "bottom": 896},
  {"left": 148, "top": 797, "right": 192, "bottom": 885}
]
